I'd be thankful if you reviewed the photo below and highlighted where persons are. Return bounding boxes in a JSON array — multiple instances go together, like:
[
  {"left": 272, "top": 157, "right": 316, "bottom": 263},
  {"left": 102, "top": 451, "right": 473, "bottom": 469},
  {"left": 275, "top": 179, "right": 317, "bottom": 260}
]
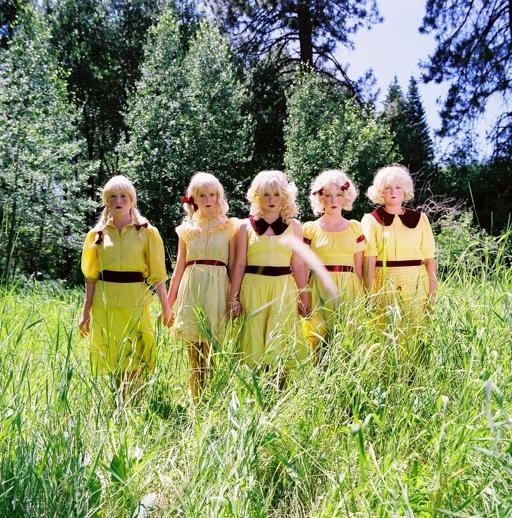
[
  {"left": 163, "top": 171, "right": 242, "bottom": 410},
  {"left": 227, "top": 169, "right": 310, "bottom": 393},
  {"left": 78, "top": 173, "right": 175, "bottom": 405},
  {"left": 361, "top": 163, "right": 441, "bottom": 361},
  {"left": 297, "top": 169, "right": 367, "bottom": 371}
]
